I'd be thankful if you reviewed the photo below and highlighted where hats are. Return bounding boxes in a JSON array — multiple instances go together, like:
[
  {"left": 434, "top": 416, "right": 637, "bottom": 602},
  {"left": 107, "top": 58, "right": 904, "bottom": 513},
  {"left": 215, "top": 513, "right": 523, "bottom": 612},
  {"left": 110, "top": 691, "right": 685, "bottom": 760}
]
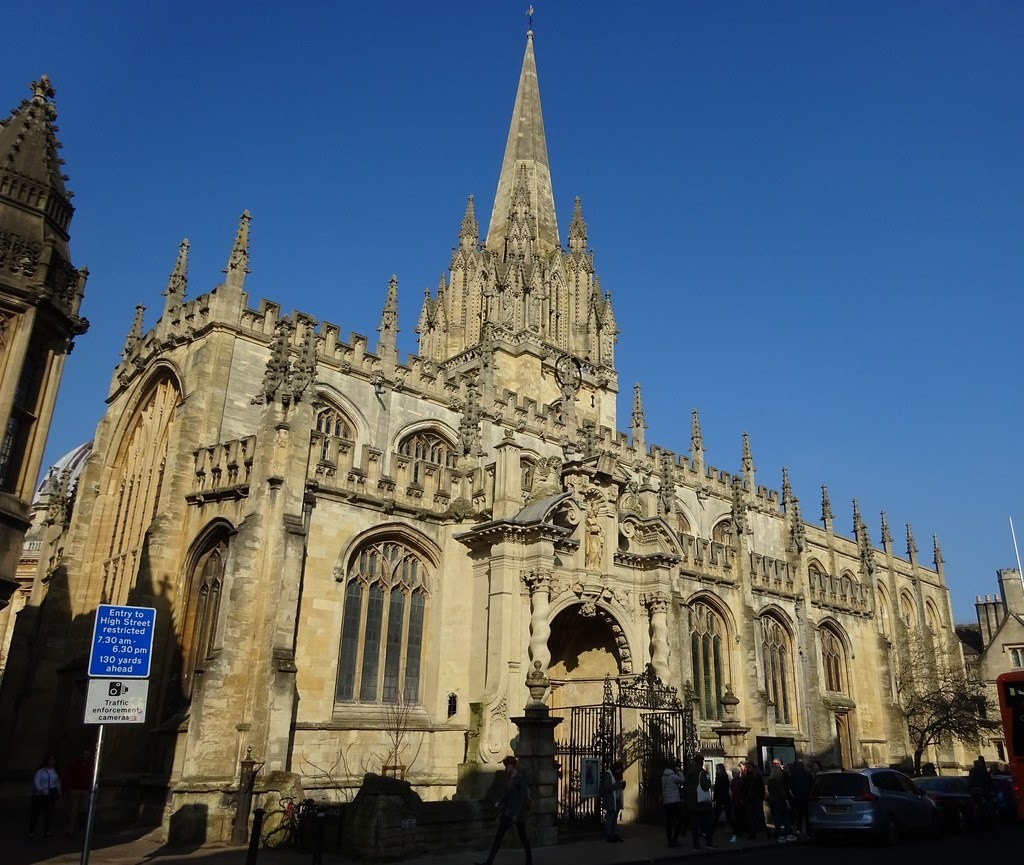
[{"left": 503, "top": 756, "right": 517, "bottom": 766}]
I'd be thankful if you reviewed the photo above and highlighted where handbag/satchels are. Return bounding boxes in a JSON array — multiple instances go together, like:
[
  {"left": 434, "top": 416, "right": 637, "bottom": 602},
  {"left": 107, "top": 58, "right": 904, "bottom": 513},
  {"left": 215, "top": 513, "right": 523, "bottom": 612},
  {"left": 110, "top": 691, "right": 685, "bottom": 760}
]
[
  {"left": 658, "top": 795, "right": 664, "bottom": 809},
  {"left": 48, "top": 787, "right": 61, "bottom": 800},
  {"left": 696, "top": 771, "right": 712, "bottom": 803}
]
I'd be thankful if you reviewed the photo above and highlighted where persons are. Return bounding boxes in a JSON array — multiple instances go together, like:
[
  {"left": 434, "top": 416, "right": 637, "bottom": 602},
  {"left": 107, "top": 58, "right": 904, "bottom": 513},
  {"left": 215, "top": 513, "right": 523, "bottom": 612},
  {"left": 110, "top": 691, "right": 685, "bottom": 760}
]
[
  {"left": 662, "top": 755, "right": 824, "bottom": 850},
  {"left": 474, "top": 756, "right": 533, "bottom": 865},
  {"left": 28, "top": 750, "right": 93, "bottom": 837},
  {"left": 988, "top": 764, "right": 1011, "bottom": 776},
  {"left": 967, "top": 756, "right": 996, "bottom": 840},
  {"left": 602, "top": 761, "right": 624, "bottom": 843}
]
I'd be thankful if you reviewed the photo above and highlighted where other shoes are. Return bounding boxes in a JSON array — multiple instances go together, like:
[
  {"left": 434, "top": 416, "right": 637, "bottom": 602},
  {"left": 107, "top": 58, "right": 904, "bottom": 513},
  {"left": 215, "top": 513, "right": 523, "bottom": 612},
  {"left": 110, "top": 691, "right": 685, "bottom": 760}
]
[
  {"left": 707, "top": 844, "right": 719, "bottom": 850},
  {"left": 28, "top": 833, "right": 35, "bottom": 839},
  {"left": 66, "top": 832, "right": 74, "bottom": 838},
  {"left": 667, "top": 842, "right": 683, "bottom": 848},
  {"left": 474, "top": 862, "right": 486, "bottom": 865},
  {"left": 730, "top": 836, "right": 738, "bottom": 844},
  {"left": 748, "top": 833, "right": 756, "bottom": 841},
  {"left": 778, "top": 836, "right": 786, "bottom": 843},
  {"left": 797, "top": 830, "right": 801, "bottom": 835},
  {"left": 607, "top": 834, "right": 624, "bottom": 843},
  {"left": 786, "top": 835, "right": 797, "bottom": 841},
  {"left": 693, "top": 844, "right": 700, "bottom": 851},
  {"left": 44, "top": 832, "right": 53, "bottom": 838}
]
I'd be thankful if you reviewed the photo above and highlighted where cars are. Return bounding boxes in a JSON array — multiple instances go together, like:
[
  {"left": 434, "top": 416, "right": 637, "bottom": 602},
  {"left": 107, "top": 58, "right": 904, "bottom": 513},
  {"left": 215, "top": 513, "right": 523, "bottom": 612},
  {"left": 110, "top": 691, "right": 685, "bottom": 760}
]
[
  {"left": 911, "top": 774, "right": 1014, "bottom": 835},
  {"left": 806, "top": 767, "right": 935, "bottom": 848}
]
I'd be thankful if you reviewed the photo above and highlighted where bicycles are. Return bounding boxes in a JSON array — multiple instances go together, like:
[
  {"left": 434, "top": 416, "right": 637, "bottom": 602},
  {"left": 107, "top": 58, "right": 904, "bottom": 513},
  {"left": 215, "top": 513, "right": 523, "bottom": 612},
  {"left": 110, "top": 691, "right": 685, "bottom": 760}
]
[{"left": 260, "top": 796, "right": 318, "bottom": 855}]
